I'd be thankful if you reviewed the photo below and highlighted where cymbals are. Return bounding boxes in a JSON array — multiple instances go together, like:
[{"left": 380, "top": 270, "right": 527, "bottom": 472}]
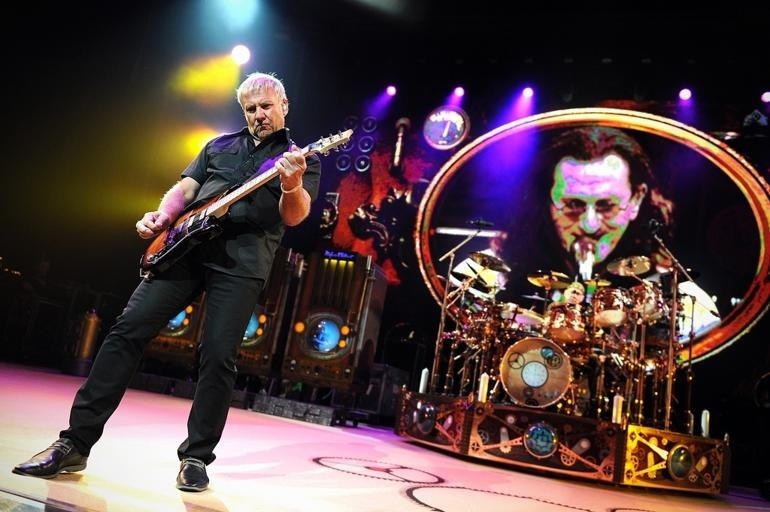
[
  {"left": 528, "top": 270, "right": 571, "bottom": 288},
  {"left": 468, "top": 251, "right": 512, "bottom": 273},
  {"left": 586, "top": 279, "right": 611, "bottom": 287},
  {"left": 606, "top": 256, "right": 652, "bottom": 276},
  {"left": 450, "top": 270, "right": 492, "bottom": 292},
  {"left": 466, "top": 263, "right": 488, "bottom": 284}
]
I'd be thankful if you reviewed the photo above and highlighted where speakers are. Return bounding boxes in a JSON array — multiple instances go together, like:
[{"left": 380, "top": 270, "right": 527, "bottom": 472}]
[
  {"left": 148, "top": 290, "right": 207, "bottom": 362},
  {"left": 282, "top": 244, "right": 389, "bottom": 391},
  {"left": 232, "top": 248, "right": 299, "bottom": 380}
]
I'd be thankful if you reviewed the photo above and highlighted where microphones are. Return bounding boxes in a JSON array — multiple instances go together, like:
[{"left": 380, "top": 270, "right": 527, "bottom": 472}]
[
  {"left": 648, "top": 219, "right": 660, "bottom": 232},
  {"left": 466, "top": 219, "right": 495, "bottom": 227}
]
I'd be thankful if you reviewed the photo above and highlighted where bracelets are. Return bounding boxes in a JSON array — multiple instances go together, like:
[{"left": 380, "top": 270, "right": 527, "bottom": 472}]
[{"left": 280, "top": 183, "right": 303, "bottom": 194}]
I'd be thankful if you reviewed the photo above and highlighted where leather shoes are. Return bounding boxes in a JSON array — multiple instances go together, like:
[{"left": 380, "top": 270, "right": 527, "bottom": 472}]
[
  {"left": 176, "top": 458, "right": 209, "bottom": 492},
  {"left": 12, "top": 439, "right": 87, "bottom": 480}
]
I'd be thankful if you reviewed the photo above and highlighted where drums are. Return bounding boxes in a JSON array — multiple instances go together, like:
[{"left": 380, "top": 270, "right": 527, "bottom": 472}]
[
  {"left": 500, "top": 337, "right": 571, "bottom": 408},
  {"left": 626, "top": 282, "right": 669, "bottom": 325},
  {"left": 591, "top": 289, "right": 631, "bottom": 327},
  {"left": 541, "top": 302, "right": 591, "bottom": 349},
  {"left": 467, "top": 319, "right": 488, "bottom": 348}
]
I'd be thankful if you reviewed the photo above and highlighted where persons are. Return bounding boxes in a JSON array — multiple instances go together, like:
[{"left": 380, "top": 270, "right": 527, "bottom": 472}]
[
  {"left": 12, "top": 71, "right": 324, "bottom": 491},
  {"left": 471, "top": 125, "right": 720, "bottom": 348}
]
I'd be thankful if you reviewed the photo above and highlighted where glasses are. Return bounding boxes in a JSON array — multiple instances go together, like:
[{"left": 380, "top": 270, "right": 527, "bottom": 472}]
[{"left": 548, "top": 186, "right": 638, "bottom": 216}]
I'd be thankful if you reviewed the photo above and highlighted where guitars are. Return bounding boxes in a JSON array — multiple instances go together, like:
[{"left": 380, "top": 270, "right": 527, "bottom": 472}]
[{"left": 139, "top": 129, "right": 354, "bottom": 280}]
